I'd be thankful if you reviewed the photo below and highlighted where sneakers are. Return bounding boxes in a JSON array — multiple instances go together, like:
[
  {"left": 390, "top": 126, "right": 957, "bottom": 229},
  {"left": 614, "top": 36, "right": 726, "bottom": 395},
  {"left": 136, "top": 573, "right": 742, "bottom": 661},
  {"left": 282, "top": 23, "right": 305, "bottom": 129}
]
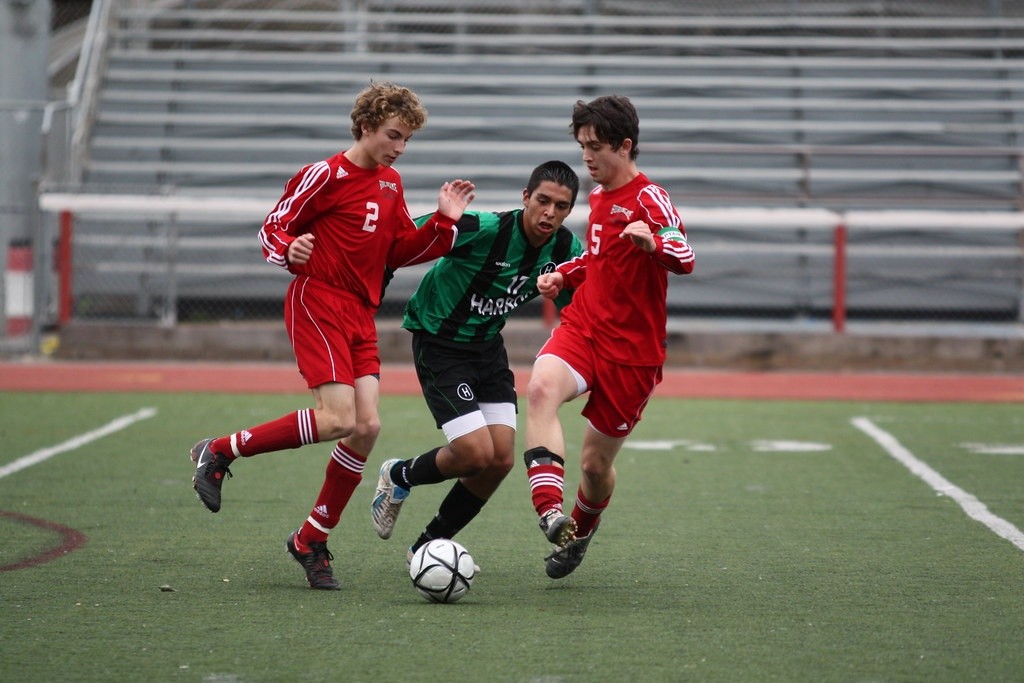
[
  {"left": 371, "top": 458, "right": 410, "bottom": 540},
  {"left": 189, "top": 437, "right": 232, "bottom": 513},
  {"left": 539, "top": 508, "right": 578, "bottom": 548},
  {"left": 287, "top": 527, "right": 341, "bottom": 590},
  {"left": 544, "top": 518, "right": 600, "bottom": 580},
  {"left": 406, "top": 546, "right": 481, "bottom": 574}
]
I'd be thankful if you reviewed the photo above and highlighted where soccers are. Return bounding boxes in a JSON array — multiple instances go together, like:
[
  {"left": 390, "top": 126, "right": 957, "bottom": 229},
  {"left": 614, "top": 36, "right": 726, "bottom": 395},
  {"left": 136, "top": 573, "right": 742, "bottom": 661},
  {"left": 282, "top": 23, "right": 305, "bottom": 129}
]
[{"left": 407, "top": 537, "right": 477, "bottom": 606}]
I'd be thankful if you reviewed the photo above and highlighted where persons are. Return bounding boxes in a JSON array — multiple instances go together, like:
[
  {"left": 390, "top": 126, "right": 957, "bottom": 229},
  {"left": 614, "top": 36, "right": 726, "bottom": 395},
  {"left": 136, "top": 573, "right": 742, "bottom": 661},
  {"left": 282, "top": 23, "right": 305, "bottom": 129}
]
[
  {"left": 371, "top": 159, "right": 586, "bottom": 574},
  {"left": 188, "top": 87, "right": 476, "bottom": 591},
  {"left": 523, "top": 98, "right": 697, "bottom": 579}
]
